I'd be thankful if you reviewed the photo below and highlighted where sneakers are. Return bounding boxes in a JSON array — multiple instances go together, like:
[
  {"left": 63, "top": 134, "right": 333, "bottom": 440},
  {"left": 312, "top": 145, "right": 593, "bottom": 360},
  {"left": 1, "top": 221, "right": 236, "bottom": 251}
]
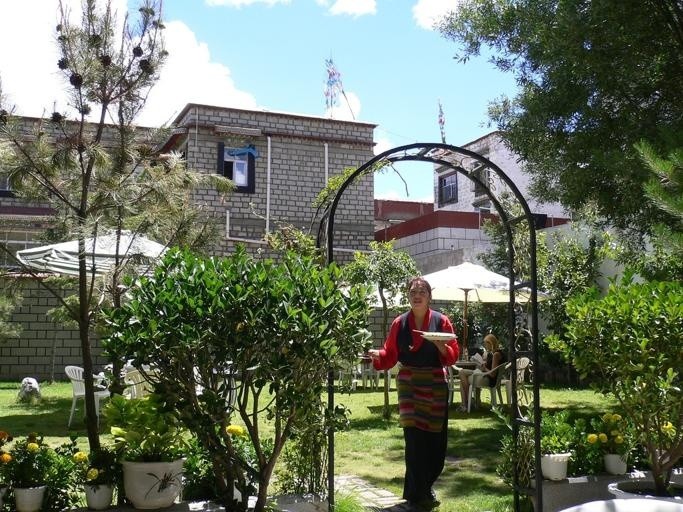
[
  {"left": 456, "top": 406, "right": 468, "bottom": 412},
  {"left": 406, "top": 498, "right": 432, "bottom": 510},
  {"left": 426, "top": 488, "right": 436, "bottom": 503}
]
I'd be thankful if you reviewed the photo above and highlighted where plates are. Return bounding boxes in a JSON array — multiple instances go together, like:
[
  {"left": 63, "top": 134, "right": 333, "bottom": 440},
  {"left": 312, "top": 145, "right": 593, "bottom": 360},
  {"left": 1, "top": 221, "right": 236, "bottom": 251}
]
[{"left": 418, "top": 332, "right": 457, "bottom": 341}]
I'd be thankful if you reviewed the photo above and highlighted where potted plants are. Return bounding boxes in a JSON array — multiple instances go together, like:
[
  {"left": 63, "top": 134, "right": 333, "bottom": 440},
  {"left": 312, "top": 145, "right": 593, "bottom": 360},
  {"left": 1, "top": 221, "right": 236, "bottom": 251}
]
[
  {"left": 110, "top": 395, "right": 193, "bottom": 507},
  {"left": 545, "top": 273, "right": 683, "bottom": 502},
  {"left": 498, "top": 411, "right": 571, "bottom": 484}
]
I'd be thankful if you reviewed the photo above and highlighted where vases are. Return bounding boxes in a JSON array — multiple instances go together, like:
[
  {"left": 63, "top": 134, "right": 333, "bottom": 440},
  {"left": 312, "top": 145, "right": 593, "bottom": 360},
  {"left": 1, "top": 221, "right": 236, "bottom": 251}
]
[
  {"left": 12, "top": 484, "right": 48, "bottom": 511},
  {"left": 85, "top": 482, "right": 111, "bottom": 509},
  {"left": 603, "top": 454, "right": 629, "bottom": 475}
]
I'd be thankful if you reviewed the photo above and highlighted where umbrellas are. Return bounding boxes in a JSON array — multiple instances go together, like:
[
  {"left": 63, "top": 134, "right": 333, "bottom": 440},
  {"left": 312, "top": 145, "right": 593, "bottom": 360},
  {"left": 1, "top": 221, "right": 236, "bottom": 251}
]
[
  {"left": 15, "top": 229, "right": 206, "bottom": 280},
  {"left": 339, "top": 262, "right": 549, "bottom": 361}
]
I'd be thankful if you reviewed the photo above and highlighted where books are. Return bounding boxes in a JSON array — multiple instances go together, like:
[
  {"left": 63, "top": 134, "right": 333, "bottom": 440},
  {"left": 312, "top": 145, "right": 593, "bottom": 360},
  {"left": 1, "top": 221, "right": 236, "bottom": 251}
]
[{"left": 470, "top": 352, "right": 484, "bottom": 367}]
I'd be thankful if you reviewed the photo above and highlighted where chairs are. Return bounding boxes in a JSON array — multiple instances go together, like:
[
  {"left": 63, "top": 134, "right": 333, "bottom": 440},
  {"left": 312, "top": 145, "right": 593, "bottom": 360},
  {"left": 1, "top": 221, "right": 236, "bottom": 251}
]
[
  {"left": 448, "top": 358, "right": 530, "bottom": 411},
  {"left": 122, "top": 367, "right": 166, "bottom": 402},
  {"left": 64, "top": 365, "right": 107, "bottom": 429},
  {"left": 337, "top": 352, "right": 381, "bottom": 392}
]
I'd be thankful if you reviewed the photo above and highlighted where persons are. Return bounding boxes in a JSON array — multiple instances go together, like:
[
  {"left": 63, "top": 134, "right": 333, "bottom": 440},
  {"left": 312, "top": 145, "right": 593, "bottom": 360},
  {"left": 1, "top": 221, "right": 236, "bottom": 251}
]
[
  {"left": 367, "top": 278, "right": 460, "bottom": 511},
  {"left": 456, "top": 334, "right": 501, "bottom": 413}
]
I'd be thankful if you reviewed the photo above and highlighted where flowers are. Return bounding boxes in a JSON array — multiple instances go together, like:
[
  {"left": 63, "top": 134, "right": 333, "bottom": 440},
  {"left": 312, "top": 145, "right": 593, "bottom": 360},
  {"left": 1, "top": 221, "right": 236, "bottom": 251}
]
[
  {"left": 0, "top": 432, "right": 74, "bottom": 512},
  {"left": 586, "top": 412, "right": 631, "bottom": 471},
  {"left": 72, "top": 448, "right": 117, "bottom": 485}
]
